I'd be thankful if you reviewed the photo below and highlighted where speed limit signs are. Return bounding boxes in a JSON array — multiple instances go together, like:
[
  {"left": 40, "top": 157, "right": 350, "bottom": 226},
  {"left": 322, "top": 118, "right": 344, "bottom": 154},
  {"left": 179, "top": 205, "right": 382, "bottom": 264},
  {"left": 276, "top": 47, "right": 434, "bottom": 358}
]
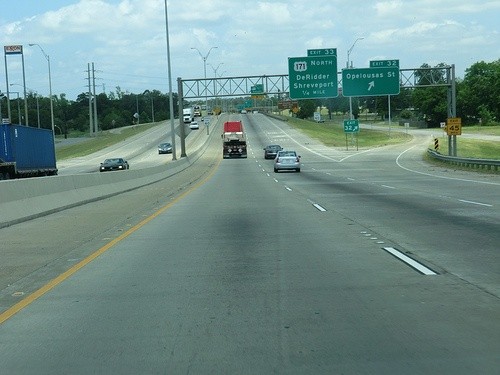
[{"left": 447, "top": 119, "right": 462, "bottom": 136}]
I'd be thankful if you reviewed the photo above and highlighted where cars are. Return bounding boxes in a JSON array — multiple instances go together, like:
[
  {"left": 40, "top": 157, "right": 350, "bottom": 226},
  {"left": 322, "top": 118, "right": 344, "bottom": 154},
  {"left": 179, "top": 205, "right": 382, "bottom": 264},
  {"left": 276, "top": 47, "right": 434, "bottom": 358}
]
[
  {"left": 158, "top": 144, "right": 173, "bottom": 154},
  {"left": 98, "top": 158, "right": 129, "bottom": 172},
  {"left": 241, "top": 110, "right": 247, "bottom": 114},
  {"left": 273, "top": 150, "right": 302, "bottom": 173},
  {"left": 182, "top": 104, "right": 214, "bottom": 130},
  {"left": 264, "top": 145, "right": 283, "bottom": 160}
]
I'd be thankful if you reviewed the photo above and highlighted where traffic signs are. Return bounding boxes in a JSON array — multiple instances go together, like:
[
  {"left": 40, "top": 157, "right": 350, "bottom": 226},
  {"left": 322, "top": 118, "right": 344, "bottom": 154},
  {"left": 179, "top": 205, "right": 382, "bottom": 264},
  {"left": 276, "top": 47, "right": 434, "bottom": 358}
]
[
  {"left": 342, "top": 60, "right": 400, "bottom": 98},
  {"left": 251, "top": 84, "right": 265, "bottom": 100},
  {"left": 345, "top": 120, "right": 360, "bottom": 133},
  {"left": 288, "top": 48, "right": 338, "bottom": 99}
]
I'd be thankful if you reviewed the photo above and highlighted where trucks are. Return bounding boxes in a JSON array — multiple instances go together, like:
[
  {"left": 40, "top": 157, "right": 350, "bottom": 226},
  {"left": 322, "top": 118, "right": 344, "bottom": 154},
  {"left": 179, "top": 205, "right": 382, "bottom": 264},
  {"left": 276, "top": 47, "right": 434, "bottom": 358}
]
[
  {"left": 221, "top": 121, "right": 248, "bottom": 158},
  {"left": 314, "top": 112, "right": 325, "bottom": 123},
  {"left": 0, "top": 123, "right": 59, "bottom": 178}
]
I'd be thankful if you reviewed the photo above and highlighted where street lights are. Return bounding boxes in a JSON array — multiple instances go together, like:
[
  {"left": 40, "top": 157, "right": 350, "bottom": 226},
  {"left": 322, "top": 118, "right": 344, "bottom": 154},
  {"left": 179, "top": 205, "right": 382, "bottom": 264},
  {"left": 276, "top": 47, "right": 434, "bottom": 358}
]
[
  {"left": 190, "top": 47, "right": 225, "bottom": 114},
  {"left": 136, "top": 96, "right": 156, "bottom": 123},
  {"left": 10, "top": 83, "right": 41, "bottom": 128},
  {"left": 28, "top": 43, "right": 58, "bottom": 162},
  {"left": 346, "top": 37, "right": 364, "bottom": 69}
]
